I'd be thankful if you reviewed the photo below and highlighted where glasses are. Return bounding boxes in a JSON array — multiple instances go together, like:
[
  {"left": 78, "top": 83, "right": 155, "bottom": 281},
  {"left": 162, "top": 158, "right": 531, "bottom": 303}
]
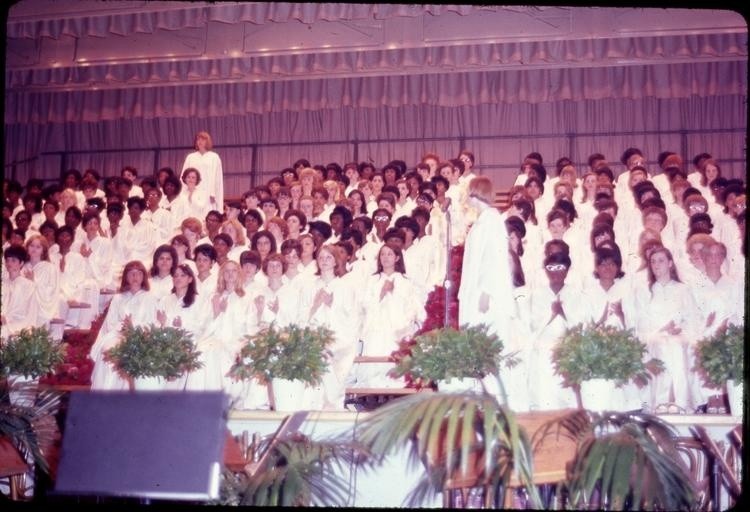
[
  {"left": 374, "top": 216, "right": 388, "bottom": 222},
  {"left": 546, "top": 265, "right": 565, "bottom": 272}
]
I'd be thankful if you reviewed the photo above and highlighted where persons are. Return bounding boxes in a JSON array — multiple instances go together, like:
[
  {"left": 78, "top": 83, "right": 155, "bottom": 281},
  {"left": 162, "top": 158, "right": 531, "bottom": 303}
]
[
  {"left": 0, "top": 145, "right": 748, "bottom": 415},
  {"left": 179, "top": 131, "right": 225, "bottom": 216}
]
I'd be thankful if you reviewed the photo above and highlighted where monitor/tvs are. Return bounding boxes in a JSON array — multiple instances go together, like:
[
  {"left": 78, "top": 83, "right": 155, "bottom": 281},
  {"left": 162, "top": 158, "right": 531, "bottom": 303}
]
[{"left": 54, "top": 390, "right": 229, "bottom": 504}]
